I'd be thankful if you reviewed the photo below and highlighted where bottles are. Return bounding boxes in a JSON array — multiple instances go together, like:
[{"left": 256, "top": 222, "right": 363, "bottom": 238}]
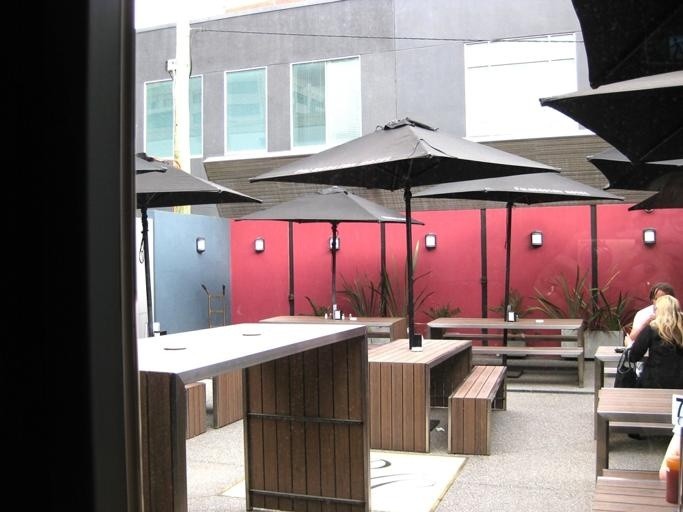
[{"left": 666, "top": 449, "right": 680, "bottom": 503}]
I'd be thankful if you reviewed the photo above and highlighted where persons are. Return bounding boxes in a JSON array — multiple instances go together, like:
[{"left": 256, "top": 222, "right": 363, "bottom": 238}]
[
  {"left": 625, "top": 295, "right": 683, "bottom": 440},
  {"left": 630, "top": 283, "right": 675, "bottom": 378},
  {"left": 658, "top": 425, "right": 681, "bottom": 479}
]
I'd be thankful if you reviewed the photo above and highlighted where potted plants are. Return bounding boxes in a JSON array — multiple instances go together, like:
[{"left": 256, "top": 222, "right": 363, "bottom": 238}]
[{"left": 488, "top": 265, "right": 645, "bottom": 357}]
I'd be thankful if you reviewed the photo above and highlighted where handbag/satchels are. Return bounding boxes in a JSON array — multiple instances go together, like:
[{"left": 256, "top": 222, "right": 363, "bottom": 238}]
[{"left": 614, "top": 348, "right": 637, "bottom": 388}]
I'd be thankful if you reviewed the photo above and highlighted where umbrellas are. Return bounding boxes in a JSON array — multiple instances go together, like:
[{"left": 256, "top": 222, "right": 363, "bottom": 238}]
[
  {"left": 540, "top": 0, "right": 683, "bottom": 211},
  {"left": 136, "top": 153, "right": 262, "bottom": 337},
  {"left": 412, "top": 172, "right": 624, "bottom": 366},
  {"left": 234, "top": 186, "right": 425, "bottom": 319},
  {"left": 249, "top": 117, "right": 561, "bottom": 349}
]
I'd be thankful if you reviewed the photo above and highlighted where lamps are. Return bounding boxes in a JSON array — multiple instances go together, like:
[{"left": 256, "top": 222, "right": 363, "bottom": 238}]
[
  {"left": 254, "top": 238, "right": 266, "bottom": 252},
  {"left": 644, "top": 228, "right": 657, "bottom": 245},
  {"left": 531, "top": 229, "right": 542, "bottom": 246},
  {"left": 195, "top": 236, "right": 206, "bottom": 252},
  {"left": 424, "top": 232, "right": 438, "bottom": 249}
]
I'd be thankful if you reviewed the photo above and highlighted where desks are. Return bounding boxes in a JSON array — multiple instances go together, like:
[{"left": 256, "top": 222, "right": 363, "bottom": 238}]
[
  {"left": 368, "top": 339, "right": 473, "bottom": 454},
  {"left": 259, "top": 314, "right": 585, "bottom": 455},
  {"left": 139, "top": 319, "right": 373, "bottom": 512},
  {"left": 426, "top": 318, "right": 584, "bottom": 347},
  {"left": 263, "top": 316, "right": 408, "bottom": 338},
  {"left": 592, "top": 344, "right": 683, "bottom": 480}
]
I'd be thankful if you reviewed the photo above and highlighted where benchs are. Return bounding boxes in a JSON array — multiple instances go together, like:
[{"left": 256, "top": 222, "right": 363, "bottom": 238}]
[
  {"left": 473, "top": 346, "right": 584, "bottom": 386},
  {"left": 592, "top": 475, "right": 683, "bottom": 512},
  {"left": 448, "top": 366, "right": 507, "bottom": 455}
]
